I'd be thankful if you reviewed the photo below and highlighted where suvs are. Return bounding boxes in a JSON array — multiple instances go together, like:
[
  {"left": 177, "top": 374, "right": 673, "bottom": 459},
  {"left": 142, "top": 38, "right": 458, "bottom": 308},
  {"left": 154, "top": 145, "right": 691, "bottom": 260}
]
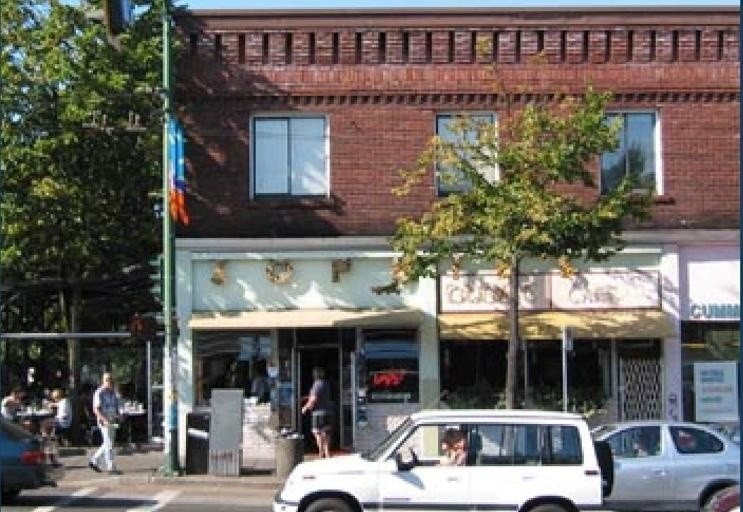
[{"left": 266, "top": 406, "right": 616, "bottom": 510}]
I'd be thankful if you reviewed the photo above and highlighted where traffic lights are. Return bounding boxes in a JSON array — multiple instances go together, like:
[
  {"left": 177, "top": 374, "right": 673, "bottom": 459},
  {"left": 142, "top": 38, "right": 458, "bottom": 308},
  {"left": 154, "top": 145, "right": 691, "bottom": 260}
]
[{"left": 132, "top": 314, "right": 157, "bottom": 342}]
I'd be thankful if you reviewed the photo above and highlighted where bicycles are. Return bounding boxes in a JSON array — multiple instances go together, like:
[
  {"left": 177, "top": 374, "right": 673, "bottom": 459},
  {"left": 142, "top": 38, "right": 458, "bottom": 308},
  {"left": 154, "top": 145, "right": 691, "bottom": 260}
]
[{"left": 147, "top": 255, "right": 162, "bottom": 302}]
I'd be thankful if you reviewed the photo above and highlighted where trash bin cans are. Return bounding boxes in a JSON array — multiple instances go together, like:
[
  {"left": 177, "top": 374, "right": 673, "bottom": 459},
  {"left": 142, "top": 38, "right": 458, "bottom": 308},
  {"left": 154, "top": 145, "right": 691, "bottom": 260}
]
[
  {"left": 186, "top": 412, "right": 210, "bottom": 475},
  {"left": 275, "top": 437, "right": 305, "bottom": 481}
]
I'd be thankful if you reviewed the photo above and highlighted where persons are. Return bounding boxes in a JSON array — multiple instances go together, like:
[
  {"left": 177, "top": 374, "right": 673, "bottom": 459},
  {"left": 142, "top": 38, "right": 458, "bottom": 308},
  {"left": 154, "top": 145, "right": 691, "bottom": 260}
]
[
  {"left": 451, "top": 431, "right": 468, "bottom": 465},
  {"left": 0, "top": 383, "right": 98, "bottom": 448},
  {"left": 301, "top": 366, "right": 332, "bottom": 458},
  {"left": 633, "top": 436, "right": 649, "bottom": 458},
  {"left": 441, "top": 439, "right": 454, "bottom": 458},
  {"left": 250, "top": 376, "right": 270, "bottom": 404},
  {"left": 89, "top": 372, "right": 123, "bottom": 475}
]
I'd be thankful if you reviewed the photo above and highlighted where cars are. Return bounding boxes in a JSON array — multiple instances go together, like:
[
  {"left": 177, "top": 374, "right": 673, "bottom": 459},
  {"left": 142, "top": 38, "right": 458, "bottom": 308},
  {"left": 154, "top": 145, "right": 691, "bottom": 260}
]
[
  {"left": 576, "top": 418, "right": 741, "bottom": 505},
  {"left": 6, "top": 393, "right": 94, "bottom": 448},
  {"left": 0, "top": 409, "right": 66, "bottom": 506},
  {"left": 341, "top": 338, "right": 452, "bottom": 402}
]
